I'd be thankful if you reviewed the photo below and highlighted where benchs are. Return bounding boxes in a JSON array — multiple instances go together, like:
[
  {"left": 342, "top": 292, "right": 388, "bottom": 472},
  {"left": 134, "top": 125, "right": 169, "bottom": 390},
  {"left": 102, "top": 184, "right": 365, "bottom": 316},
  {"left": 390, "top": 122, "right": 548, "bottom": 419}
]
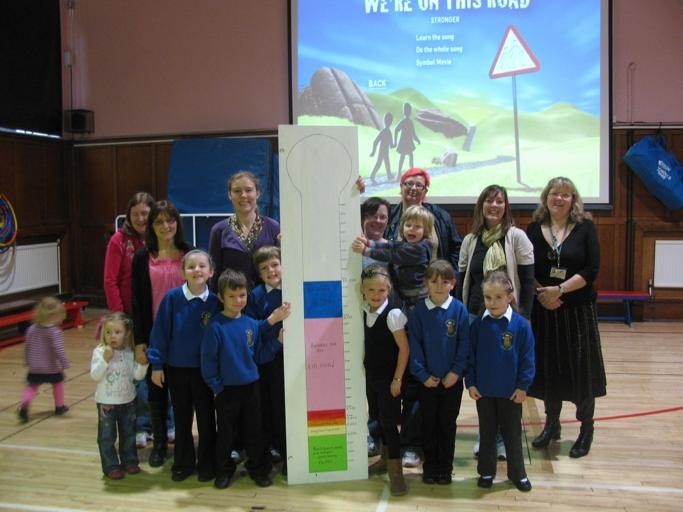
[
  {"left": 0, "top": 300, "right": 89, "bottom": 348},
  {"left": 596, "top": 291, "right": 650, "bottom": 327}
]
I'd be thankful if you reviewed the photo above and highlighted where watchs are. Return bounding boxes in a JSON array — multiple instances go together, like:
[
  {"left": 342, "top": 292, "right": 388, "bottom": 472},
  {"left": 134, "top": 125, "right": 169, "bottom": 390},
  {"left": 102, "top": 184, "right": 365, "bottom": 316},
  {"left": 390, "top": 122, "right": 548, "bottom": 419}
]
[{"left": 558, "top": 285, "right": 567, "bottom": 295}]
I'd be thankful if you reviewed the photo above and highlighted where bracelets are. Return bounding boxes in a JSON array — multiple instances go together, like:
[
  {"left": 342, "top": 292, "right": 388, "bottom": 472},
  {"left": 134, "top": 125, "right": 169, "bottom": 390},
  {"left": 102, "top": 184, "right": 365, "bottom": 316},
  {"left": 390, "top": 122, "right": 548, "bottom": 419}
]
[{"left": 391, "top": 377, "right": 402, "bottom": 384}]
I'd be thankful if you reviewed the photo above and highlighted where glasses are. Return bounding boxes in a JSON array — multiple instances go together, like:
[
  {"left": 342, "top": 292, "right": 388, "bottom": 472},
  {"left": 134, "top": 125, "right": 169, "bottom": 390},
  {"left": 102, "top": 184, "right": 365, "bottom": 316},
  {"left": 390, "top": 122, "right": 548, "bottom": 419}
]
[
  {"left": 153, "top": 216, "right": 175, "bottom": 226},
  {"left": 403, "top": 181, "right": 427, "bottom": 194}
]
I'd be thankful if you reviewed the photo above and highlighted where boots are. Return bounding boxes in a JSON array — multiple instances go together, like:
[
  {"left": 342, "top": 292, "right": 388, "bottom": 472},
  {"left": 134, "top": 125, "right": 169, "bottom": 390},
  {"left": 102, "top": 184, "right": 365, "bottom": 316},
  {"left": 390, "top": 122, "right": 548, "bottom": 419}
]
[
  {"left": 387, "top": 455, "right": 408, "bottom": 496},
  {"left": 368, "top": 446, "right": 388, "bottom": 475}
]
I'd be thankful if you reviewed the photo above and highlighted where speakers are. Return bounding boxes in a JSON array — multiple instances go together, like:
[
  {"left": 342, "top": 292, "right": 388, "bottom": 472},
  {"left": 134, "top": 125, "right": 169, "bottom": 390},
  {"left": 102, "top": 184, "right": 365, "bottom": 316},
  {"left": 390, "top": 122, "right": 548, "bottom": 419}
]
[{"left": 64, "top": 109, "right": 95, "bottom": 134}]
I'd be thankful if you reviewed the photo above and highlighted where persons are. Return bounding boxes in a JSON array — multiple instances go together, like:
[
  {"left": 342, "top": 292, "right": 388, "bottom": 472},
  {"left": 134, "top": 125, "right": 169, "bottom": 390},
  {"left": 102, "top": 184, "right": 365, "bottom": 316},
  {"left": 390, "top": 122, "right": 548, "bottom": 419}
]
[
  {"left": 147, "top": 249, "right": 224, "bottom": 481},
  {"left": 394, "top": 103, "right": 420, "bottom": 169},
  {"left": 370, "top": 113, "right": 394, "bottom": 185},
  {"left": 383, "top": 168, "right": 464, "bottom": 296},
  {"left": 351, "top": 205, "right": 435, "bottom": 306},
  {"left": 200, "top": 268, "right": 284, "bottom": 488},
  {"left": 465, "top": 270, "right": 537, "bottom": 490},
  {"left": 209, "top": 172, "right": 280, "bottom": 289},
  {"left": 407, "top": 259, "right": 469, "bottom": 484},
  {"left": 90, "top": 311, "right": 150, "bottom": 479},
  {"left": 244, "top": 246, "right": 292, "bottom": 479},
  {"left": 19, "top": 296, "right": 73, "bottom": 422},
  {"left": 458, "top": 185, "right": 534, "bottom": 460},
  {"left": 132, "top": 201, "right": 195, "bottom": 465},
  {"left": 102, "top": 193, "right": 176, "bottom": 448},
  {"left": 356, "top": 175, "right": 424, "bottom": 466},
  {"left": 361, "top": 264, "right": 410, "bottom": 495},
  {"left": 526, "top": 176, "right": 607, "bottom": 457}
]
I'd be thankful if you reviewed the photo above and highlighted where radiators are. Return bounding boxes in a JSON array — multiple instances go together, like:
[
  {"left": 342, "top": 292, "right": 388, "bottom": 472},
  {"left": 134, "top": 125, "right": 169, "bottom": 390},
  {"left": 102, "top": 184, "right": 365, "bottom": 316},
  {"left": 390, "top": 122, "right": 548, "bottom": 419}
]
[
  {"left": 649, "top": 239, "right": 683, "bottom": 298},
  {"left": 0, "top": 238, "right": 62, "bottom": 296}
]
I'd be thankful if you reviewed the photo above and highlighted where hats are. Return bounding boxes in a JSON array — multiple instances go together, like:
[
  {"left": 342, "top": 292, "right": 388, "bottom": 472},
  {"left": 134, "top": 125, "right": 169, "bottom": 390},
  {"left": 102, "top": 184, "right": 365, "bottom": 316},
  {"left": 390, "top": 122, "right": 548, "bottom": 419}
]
[{"left": 36, "top": 297, "right": 67, "bottom": 322}]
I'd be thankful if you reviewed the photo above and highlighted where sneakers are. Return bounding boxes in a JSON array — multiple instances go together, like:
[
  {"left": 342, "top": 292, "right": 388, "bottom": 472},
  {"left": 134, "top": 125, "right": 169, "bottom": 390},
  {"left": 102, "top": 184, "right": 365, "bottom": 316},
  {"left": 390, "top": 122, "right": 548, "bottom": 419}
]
[
  {"left": 255, "top": 474, "right": 270, "bottom": 486},
  {"left": 136, "top": 431, "right": 147, "bottom": 448},
  {"left": 215, "top": 474, "right": 229, "bottom": 488},
  {"left": 513, "top": 479, "right": 531, "bottom": 491},
  {"left": 125, "top": 466, "right": 140, "bottom": 473},
  {"left": 270, "top": 449, "right": 281, "bottom": 458},
  {"left": 423, "top": 473, "right": 437, "bottom": 483},
  {"left": 231, "top": 450, "right": 240, "bottom": 458},
  {"left": 496, "top": 445, "right": 507, "bottom": 459},
  {"left": 368, "top": 442, "right": 380, "bottom": 456},
  {"left": 149, "top": 446, "right": 168, "bottom": 467},
  {"left": 168, "top": 432, "right": 175, "bottom": 443},
  {"left": 437, "top": 474, "right": 451, "bottom": 484},
  {"left": 172, "top": 465, "right": 195, "bottom": 481},
  {"left": 108, "top": 471, "right": 124, "bottom": 479},
  {"left": 55, "top": 405, "right": 69, "bottom": 415},
  {"left": 402, "top": 451, "right": 421, "bottom": 467},
  {"left": 478, "top": 475, "right": 494, "bottom": 488},
  {"left": 19, "top": 407, "right": 28, "bottom": 423}
]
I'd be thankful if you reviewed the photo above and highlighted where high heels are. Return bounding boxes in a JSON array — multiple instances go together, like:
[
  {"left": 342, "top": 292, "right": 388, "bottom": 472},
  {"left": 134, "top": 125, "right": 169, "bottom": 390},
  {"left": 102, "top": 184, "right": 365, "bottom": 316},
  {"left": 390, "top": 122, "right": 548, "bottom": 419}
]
[
  {"left": 532, "top": 419, "right": 561, "bottom": 447},
  {"left": 569, "top": 429, "right": 594, "bottom": 457}
]
